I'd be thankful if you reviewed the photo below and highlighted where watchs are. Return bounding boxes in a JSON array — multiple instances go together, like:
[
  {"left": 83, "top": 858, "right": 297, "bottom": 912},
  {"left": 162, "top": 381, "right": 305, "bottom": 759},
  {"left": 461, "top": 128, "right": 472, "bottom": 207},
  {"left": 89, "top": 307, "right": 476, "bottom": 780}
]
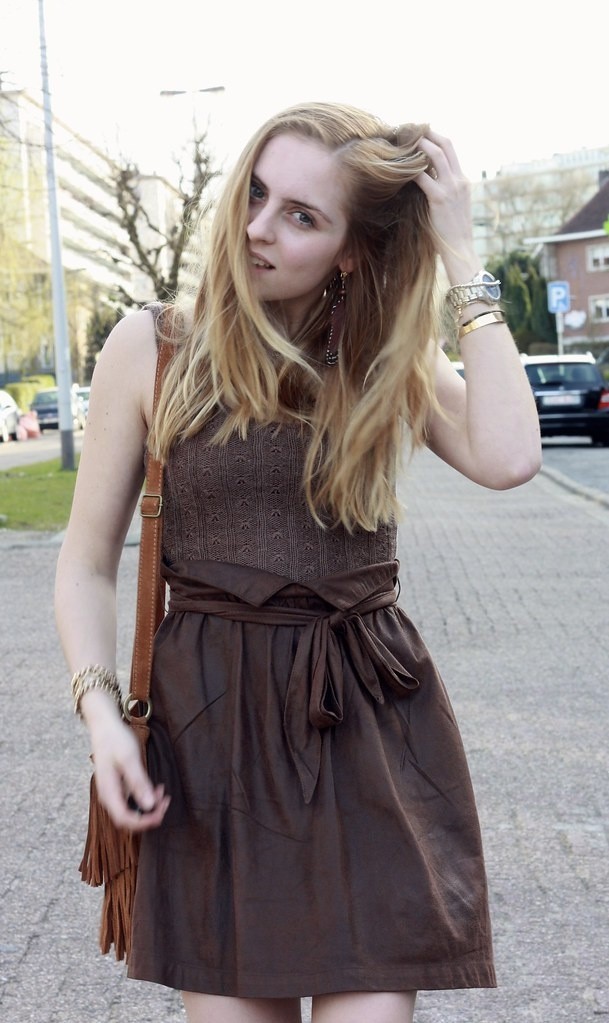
[{"left": 444, "top": 269, "right": 506, "bottom": 310}]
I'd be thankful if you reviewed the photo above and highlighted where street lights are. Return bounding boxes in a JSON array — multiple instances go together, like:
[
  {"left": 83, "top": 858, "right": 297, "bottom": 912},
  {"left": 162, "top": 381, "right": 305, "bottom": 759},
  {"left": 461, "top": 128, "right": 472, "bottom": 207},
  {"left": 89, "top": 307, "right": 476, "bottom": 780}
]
[{"left": 160, "top": 84, "right": 226, "bottom": 190}]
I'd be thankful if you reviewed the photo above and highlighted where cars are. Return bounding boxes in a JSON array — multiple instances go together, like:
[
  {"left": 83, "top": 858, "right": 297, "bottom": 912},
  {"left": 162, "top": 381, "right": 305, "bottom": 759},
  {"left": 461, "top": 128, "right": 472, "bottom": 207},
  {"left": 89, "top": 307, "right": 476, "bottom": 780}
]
[
  {"left": 0, "top": 391, "right": 25, "bottom": 443},
  {"left": 76, "top": 385, "right": 91, "bottom": 418},
  {"left": 28, "top": 387, "right": 59, "bottom": 433}
]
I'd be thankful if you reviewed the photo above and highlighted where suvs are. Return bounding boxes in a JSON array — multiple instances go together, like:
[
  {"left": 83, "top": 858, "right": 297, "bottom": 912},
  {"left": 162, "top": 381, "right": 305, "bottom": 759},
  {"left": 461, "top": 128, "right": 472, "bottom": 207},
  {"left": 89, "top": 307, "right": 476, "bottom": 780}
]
[{"left": 519, "top": 351, "right": 609, "bottom": 449}]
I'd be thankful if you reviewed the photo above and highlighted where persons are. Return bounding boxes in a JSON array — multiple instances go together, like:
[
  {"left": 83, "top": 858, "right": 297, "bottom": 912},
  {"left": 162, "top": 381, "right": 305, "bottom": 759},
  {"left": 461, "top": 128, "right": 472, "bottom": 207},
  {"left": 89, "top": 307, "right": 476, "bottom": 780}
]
[{"left": 47, "top": 100, "right": 548, "bottom": 1022}]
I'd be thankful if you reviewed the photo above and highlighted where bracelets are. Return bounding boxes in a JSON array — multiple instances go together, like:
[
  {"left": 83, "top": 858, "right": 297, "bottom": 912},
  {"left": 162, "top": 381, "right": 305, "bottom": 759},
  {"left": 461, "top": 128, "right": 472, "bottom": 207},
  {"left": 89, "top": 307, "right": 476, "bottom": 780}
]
[
  {"left": 68, "top": 663, "right": 126, "bottom": 718},
  {"left": 453, "top": 309, "right": 507, "bottom": 339}
]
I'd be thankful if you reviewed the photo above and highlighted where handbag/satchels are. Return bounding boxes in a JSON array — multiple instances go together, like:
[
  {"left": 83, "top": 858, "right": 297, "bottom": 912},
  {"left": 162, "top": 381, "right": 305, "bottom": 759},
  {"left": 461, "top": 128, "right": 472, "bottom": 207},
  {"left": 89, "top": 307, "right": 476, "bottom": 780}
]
[{"left": 78, "top": 699, "right": 151, "bottom": 964}]
